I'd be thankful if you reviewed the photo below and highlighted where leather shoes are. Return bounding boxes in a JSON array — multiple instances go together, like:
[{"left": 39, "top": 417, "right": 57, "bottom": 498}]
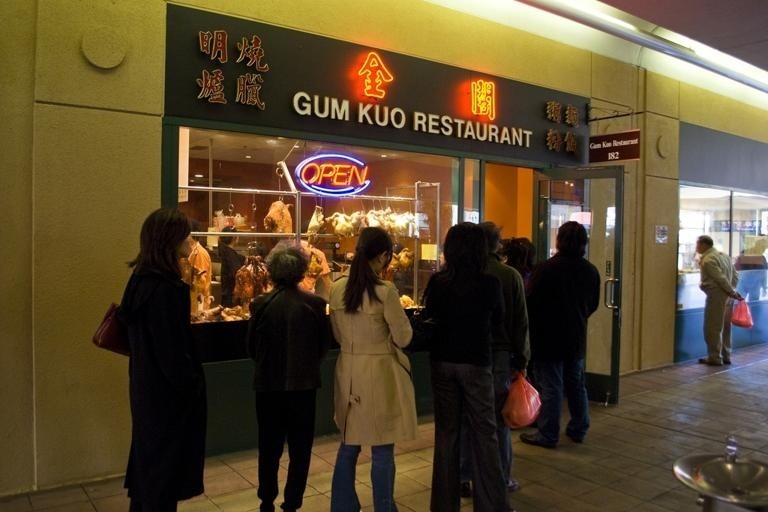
[{"left": 520, "top": 432, "right": 556, "bottom": 447}]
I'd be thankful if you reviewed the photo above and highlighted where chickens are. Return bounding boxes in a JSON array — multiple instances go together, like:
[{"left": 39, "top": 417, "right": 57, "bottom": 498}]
[
  {"left": 387, "top": 252, "right": 398, "bottom": 274},
  {"left": 325, "top": 206, "right": 416, "bottom": 244},
  {"left": 305, "top": 201, "right": 325, "bottom": 248},
  {"left": 398, "top": 248, "right": 414, "bottom": 273}
]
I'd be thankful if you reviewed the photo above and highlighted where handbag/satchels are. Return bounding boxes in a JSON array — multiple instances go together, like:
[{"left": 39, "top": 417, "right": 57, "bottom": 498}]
[
  {"left": 94, "top": 303, "right": 131, "bottom": 357},
  {"left": 410, "top": 309, "right": 433, "bottom": 338}
]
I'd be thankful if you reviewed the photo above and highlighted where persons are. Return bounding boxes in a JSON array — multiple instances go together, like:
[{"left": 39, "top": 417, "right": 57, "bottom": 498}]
[
  {"left": 696, "top": 236, "right": 744, "bottom": 366},
  {"left": 121, "top": 206, "right": 211, "bottom": 509},
  {"left": 241, "top": 246, "right": 331, "bottom": 511},
  {"left": 184, "top": 218, "right": 210, "bottom": 318},
  {"left": 520, "top": 222, "right": 600, "bottom": 452},
  {"left": 217, "top": 226, "right": 245, "bottom": 313},
  {"left": 264, "top": 220, "right": 331, "bottom": 296},
  {"left": 422, "top": 222, "right": 539, "bottom": 510},
  {"left": 329, "top": 228, "right": 420, "bottom": 510}
]
